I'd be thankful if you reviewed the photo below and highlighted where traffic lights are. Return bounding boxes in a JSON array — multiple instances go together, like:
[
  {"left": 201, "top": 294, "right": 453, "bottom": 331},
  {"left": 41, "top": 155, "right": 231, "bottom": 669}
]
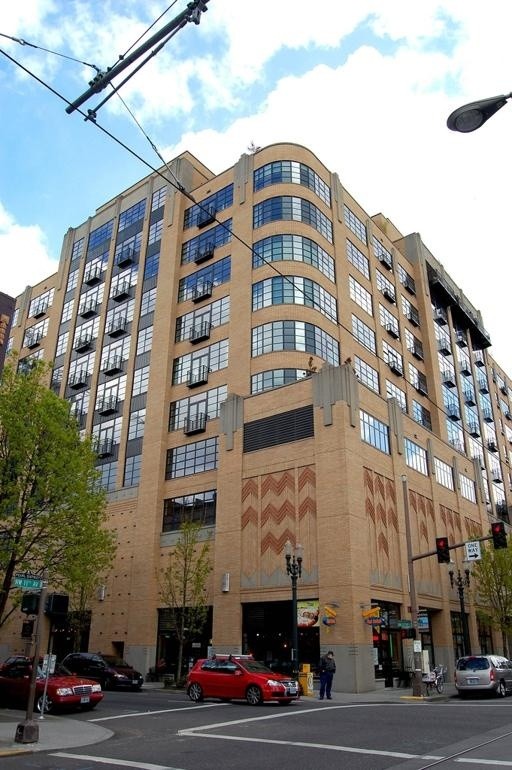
[
  {"left": 436, "top": 536, "right": 450, "bottom": 564},
  {"left": 492, "top": 521, "right": 509, "bottom": 550},
  {"left": 44, "top": 591, "right": 69, "bottom": 619},
  {"left": 20, "top": 593, "right": 41, "bottom": 615}
]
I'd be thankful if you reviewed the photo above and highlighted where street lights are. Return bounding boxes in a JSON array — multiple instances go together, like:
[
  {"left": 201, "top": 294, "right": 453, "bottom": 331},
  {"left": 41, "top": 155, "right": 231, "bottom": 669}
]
[
  {"left": 285, "top": 539, "right": 306, "bottom": 697},
  {"left": 443, "top": 88, "right": 512, "bottom": 137},
  {"left": 448, "top": 565, "right": 472, "bottom": 658}
]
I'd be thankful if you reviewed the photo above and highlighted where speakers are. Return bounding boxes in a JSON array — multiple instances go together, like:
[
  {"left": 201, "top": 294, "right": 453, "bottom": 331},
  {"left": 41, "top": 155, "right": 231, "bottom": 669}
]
[
  {"left": 222, "top": 573, "right": 230, "bottom": 592},
  {"left": 98, "top": 586, "right": 105, "bottom": 601}
]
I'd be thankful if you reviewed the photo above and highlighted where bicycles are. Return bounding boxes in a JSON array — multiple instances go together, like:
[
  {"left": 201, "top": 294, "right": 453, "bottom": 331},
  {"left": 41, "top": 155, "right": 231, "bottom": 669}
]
[{"left": 422, "top": 667, "right": 445, "bottom": 697}]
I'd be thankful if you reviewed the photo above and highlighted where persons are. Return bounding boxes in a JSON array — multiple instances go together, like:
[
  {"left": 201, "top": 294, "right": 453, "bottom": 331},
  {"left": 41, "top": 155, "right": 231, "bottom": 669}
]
[
  {"left": 307, "top": 613, "right": 315, "bottom": 627},
  {"left": 298, "top": 612, "right": 310, "bottom": 626},
  {"left": 318, "top": 650, "right": 337, "bottom": 700},
  {"left": 313, "top": 609, "right": 319, "bottom": 625}
]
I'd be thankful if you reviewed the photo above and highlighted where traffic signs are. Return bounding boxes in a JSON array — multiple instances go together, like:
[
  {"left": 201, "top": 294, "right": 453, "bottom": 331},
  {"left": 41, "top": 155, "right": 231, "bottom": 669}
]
[
  {"left": 15, "top": 572, "right": 43, "bottom": 581},
  {"left": 13, "top": 576, "right": 41, "bottom": 589},
  {"left": 465, "top": 541, "right": 484, "bottom": 560}
]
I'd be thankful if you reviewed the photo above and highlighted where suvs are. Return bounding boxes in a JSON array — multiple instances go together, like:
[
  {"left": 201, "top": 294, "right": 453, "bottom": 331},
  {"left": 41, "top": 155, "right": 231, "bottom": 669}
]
[
  {"left": 0, "top": 655, "right": 105, "bottom": 719},
  {"left": 181, "top": 652, "right": 303, "bottom": 708}
]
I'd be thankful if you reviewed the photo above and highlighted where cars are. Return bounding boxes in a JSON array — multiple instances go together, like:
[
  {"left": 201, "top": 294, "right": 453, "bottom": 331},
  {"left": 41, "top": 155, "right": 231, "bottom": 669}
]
[{"left": 57, "top": 652, "right": 144, "bottom": 692}]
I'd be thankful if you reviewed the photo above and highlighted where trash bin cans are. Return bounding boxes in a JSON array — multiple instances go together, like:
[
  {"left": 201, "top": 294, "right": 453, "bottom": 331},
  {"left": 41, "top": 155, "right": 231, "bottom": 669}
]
[{"left": 162, "top": 674, "right": 174, "bottom": 688}]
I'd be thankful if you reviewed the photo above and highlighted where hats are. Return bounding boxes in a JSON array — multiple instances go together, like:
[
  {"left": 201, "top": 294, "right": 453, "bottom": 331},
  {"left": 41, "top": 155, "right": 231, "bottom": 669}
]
[{"left": 328, "top": 651, "right": 333, "bottom": 654}]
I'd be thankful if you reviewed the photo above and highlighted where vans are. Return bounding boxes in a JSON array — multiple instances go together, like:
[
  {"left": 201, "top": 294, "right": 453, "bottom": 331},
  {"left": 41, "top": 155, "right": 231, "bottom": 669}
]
[{"left": 453, "top": 653, "right": 512, "bottom": 698}]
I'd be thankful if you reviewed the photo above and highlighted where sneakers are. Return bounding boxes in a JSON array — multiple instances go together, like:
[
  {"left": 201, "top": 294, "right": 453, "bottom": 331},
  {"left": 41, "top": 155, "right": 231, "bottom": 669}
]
[
  {"left": 327, "top": 696, "right": 332, "bottom": 699},
  {"left": 320, "top": 696, "right": 323, "bottom": 700}
]
